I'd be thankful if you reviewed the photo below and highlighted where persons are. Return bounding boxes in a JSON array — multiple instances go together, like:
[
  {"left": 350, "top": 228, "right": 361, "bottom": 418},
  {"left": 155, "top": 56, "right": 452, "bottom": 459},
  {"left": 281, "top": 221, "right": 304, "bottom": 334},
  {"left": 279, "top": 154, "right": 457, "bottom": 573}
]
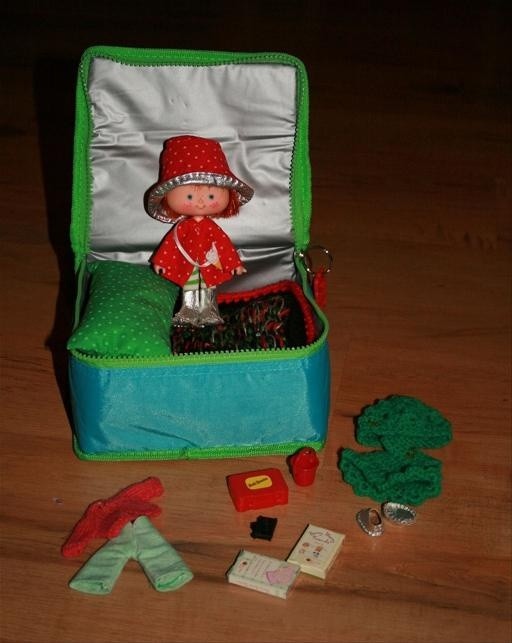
[{"left": 144, "top": 135, "right": 254, "bottom": 327}]
